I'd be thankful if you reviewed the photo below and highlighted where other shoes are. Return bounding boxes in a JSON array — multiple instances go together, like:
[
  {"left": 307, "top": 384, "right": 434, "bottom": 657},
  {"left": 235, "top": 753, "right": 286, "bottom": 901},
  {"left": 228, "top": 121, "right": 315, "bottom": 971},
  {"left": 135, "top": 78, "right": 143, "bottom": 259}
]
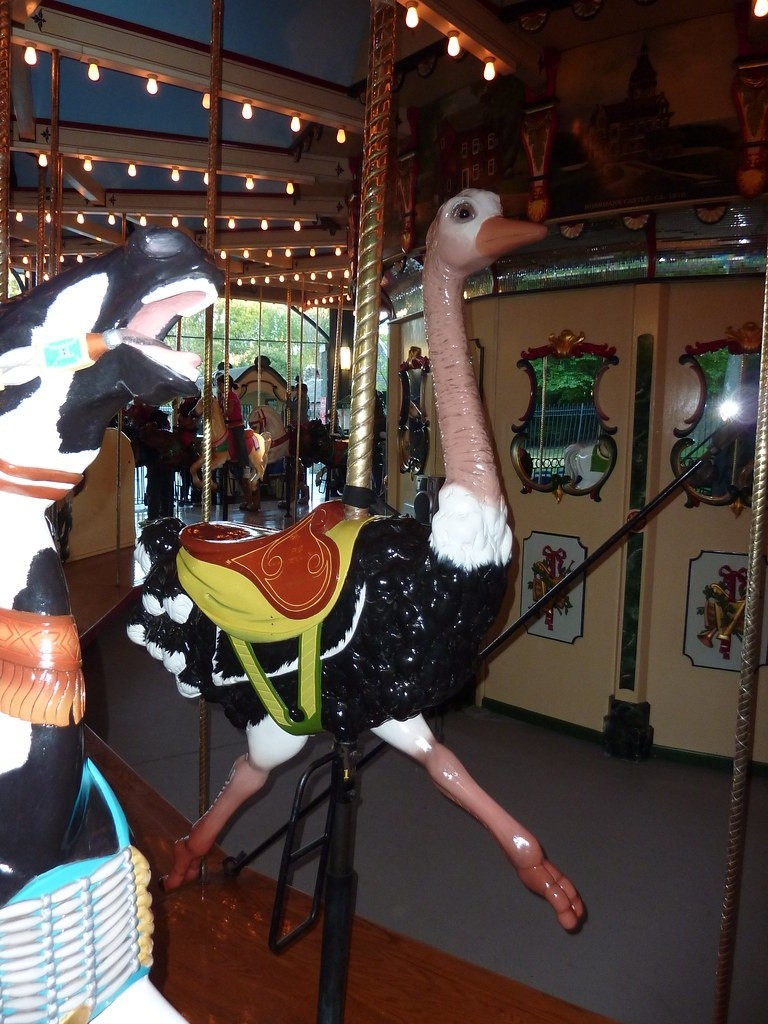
[{"left": 238, "top": 459, "right": 249, "bottom": 467}]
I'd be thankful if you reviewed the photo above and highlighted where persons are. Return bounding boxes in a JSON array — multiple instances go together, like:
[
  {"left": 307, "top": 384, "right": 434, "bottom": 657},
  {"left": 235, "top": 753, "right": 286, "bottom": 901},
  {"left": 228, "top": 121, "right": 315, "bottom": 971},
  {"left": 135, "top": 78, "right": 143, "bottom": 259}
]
[
  {"left": 284, "top": 382, "right": 311, "bottom": 451},
  {"left": 324, "top": 408, "right": 345, "bottom": 438},
  {"left": 120, "top": 394, "right": 204, "bottom": 504},
  {"left": 214, "top": 373, "right": 251, "bottom": 479}
]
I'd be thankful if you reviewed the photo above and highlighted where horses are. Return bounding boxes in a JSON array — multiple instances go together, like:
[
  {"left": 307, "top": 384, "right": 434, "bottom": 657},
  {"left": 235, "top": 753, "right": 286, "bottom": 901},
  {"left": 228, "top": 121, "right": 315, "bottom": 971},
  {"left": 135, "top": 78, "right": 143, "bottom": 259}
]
[
  {"left": 188, "top": 392, "right": 350, "bottom": 497},
  {"left": 1, "top": 224, "right": 222, "bottom": 908}
]
